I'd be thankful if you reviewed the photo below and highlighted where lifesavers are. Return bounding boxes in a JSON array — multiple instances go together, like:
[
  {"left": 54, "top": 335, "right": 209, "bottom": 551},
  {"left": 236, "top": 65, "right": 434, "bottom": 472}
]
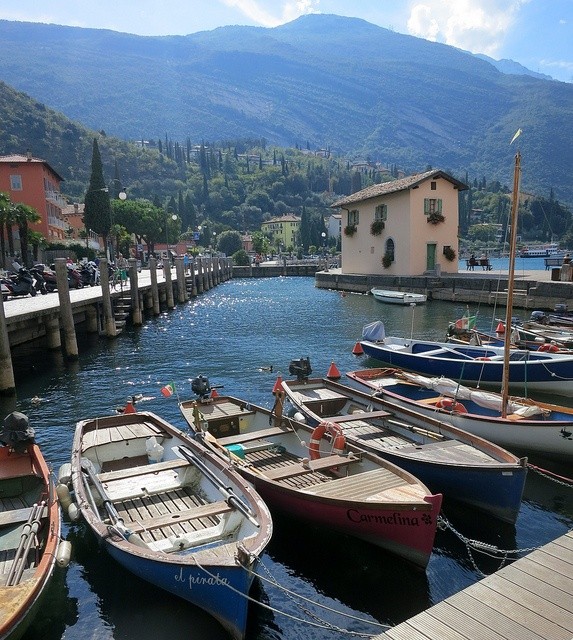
[
  {"left": 538, "top": 344, "right": 559, "bottom": 352},
  {"left": 436, "top": 397, "right": 467, "bottom": 413},
  {"left": 309, "top": 421, "right": 346, "bottom": 471}
]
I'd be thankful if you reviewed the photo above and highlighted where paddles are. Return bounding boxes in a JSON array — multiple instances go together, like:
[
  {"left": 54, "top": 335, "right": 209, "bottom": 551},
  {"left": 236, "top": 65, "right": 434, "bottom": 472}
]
[
  {"left": 12, "top": 500, "right": 47, "bottom": 586},
  {"left": 347, "top": 403, "right": 445, "bottom": 442},
  {"left": 177, "top": 444, "right": 257, "bottom": 517},
  {"left": 4, "top": 502, "right": 39, "bottom": 587},
  {"left": 203, "top": 431, "right": 270, "bottom": 480},
  {"left": 81, "top": 457, "right": 125, "bottom": 527},
  {"left": 170, "top": 445, "right": 261, "bottom": 528}
]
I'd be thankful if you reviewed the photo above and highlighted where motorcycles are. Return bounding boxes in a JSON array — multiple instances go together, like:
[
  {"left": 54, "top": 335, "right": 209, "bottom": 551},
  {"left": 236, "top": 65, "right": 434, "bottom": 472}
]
[
  {"left": 0, "top": 262, "right": 36, "bottom": 301},
  {"left": 9, "top": 268, "right": 47, "bottom": 294},
  {"left": 82, "top": 261, "right": 103, "bottom": 287},
  {"left": 34, "top": 263, "right": 83, "bottom": 292}
]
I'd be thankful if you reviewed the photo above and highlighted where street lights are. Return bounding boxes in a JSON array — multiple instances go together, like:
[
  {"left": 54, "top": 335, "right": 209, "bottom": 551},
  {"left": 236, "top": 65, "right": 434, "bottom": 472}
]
[
  {"left": 165, "top": 206, "right": 177, "bottom": 257},
  {"left": 109, "top": 179, "right": 126, "bottom": 268}
]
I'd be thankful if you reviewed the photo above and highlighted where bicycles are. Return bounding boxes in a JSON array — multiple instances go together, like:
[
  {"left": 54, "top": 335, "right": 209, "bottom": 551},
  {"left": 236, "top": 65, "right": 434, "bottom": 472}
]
[{"left": 107, "top": 268, "right": 122, "bottom": 292}]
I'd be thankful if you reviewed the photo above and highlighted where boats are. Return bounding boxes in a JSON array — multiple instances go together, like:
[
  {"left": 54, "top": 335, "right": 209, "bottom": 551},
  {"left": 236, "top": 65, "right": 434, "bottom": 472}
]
[
  {"left": 346, "top": 368, "right": 573, "bottom": 458},
  {"left": 548, "top": 303, "right": 573, "bottom": 322},
  {"left": 71, "top": 395, "right": 273, "bottom": 640},
  {"left": 370, "top": 287, "right": 426, "bottom": 305},
  {"left": 525, "top": 311, "right": 573, "bottom": 332},
  {"left": 178, "top": 375, "right": 442, "bottom": 571},
  {"left": 495, "top": 307, "right": 573, "bottom": 348},
  {"left": 280, "top": 356, "right": 527, "bottom": 525},
  {"left": 360, "top": 321, "right": 573, "bottom": 398},
  {"left": 445, "top": 321, "right": 573, "bottom": 354},
  {"left": 0, "top": 411, "right": 60, "bottom": 639}
]
